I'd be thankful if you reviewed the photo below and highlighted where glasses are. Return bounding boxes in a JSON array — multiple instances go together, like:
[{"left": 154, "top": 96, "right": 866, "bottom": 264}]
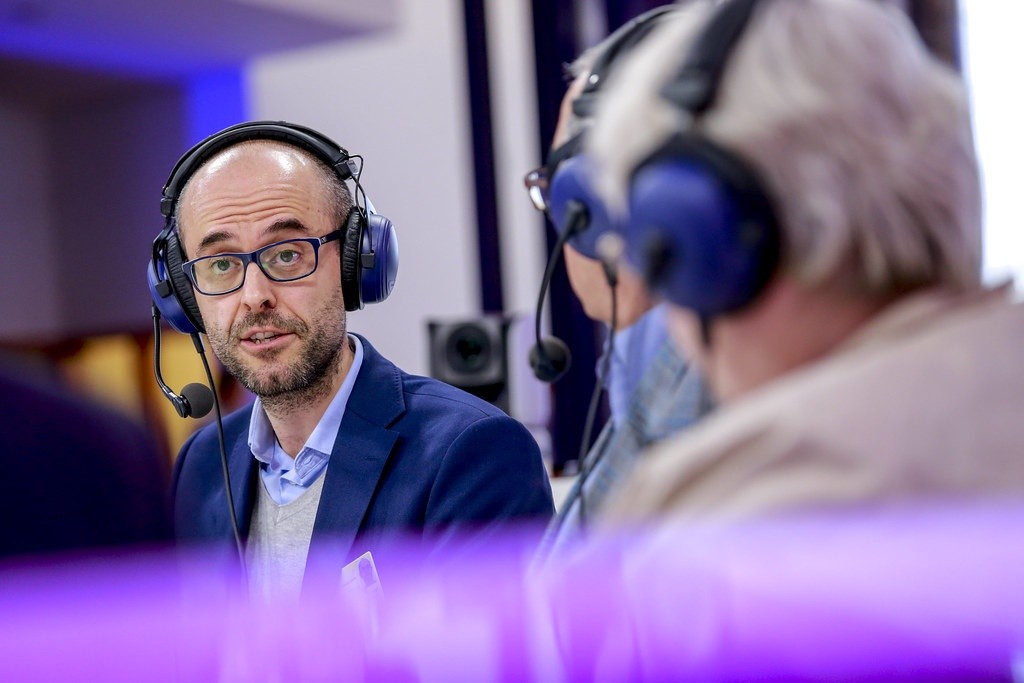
[
  {"left": 182, "top": 228, "right": 340, "bottom": 296},
  {"left": 523, "top": 167, "right": 556, "bottom": 212}
]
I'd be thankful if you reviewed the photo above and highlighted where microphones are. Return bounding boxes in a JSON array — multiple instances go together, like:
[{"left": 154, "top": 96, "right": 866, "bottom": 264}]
[
  {"left": 153, "top": 316, "right": 215, "bottom": 420},
  {"left": 528, "top": 236, "right": 569, "bottom": 384}
]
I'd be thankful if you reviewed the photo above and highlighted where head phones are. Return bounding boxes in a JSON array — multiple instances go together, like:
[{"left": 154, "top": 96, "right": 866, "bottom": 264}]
[
  {"left": 624, "top": 0, "right": 792, "bottom": 317},
  {"left": 148, "top": 121, "right": 400, "bottom": 335},
  {"left": 546, "top": 4, "right": 679, "bottom": 260}
]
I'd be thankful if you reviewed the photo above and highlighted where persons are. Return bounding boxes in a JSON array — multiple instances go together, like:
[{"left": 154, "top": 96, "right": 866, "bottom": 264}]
[
  {"left": 159, "top": 121, "right": 557, "bottom": 678},
  {"left": 521, "top": 0, "right": 1024, "bottom": 683}
]
